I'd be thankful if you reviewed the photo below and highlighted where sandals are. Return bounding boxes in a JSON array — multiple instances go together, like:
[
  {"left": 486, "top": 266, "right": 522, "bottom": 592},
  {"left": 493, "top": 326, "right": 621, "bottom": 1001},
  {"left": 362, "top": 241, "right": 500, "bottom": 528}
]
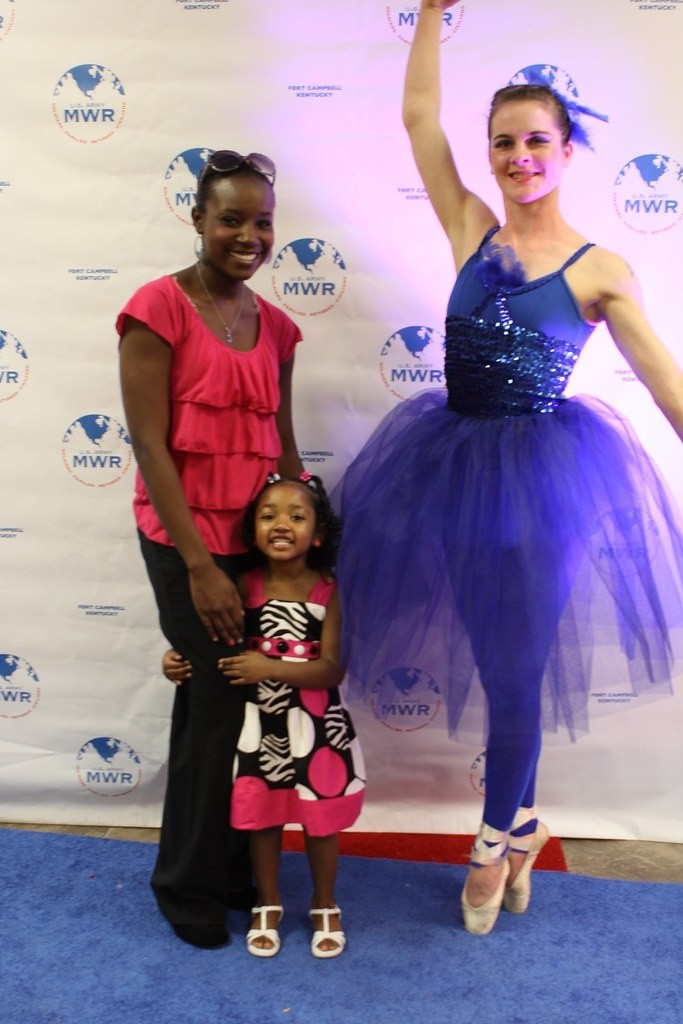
[
  {"left": 308, "top": 908, "right": 346, "bottom": 958},
  {"left": 246, "top": 902, "right": 282, "bottom": 958}
]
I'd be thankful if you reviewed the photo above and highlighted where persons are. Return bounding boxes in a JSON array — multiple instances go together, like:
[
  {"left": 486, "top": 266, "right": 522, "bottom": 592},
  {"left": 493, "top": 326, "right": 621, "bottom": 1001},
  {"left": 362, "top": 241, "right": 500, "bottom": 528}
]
[
  {"left": 333, "top": 0, "right": 683, "bottom": 934},
  {"left": 163, "top": 472, "right": 366, "bottom": 958},
  {"left": 116, "top": 150, "right": 306, "bottom": 949}
]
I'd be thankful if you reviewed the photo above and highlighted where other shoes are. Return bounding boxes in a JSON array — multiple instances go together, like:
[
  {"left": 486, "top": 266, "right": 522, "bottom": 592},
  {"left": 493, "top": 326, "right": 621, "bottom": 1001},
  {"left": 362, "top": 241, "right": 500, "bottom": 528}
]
[
  {"left": 462, "top": 824, "right": 511, "bottom": 934},
  {"left": 503, "top": 806, "right": 550, "bottom": 913},
  {"left": 222, "top": 885, "right": 259, "bottom": 911},
  {"left": 172, "top": 922, "right": 229, "bottom": 948}
]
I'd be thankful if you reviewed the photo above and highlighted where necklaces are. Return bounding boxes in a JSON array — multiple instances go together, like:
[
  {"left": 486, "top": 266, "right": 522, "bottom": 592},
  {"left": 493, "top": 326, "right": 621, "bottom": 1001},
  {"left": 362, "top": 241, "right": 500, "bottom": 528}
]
[{"left": 196, "top": 259, "right": 247, "bottom": 343}]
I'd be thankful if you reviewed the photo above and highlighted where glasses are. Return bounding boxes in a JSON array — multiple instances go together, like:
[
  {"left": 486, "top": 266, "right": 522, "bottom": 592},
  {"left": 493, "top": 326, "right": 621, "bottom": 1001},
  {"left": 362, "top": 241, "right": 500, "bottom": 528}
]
[{"left": 197, "top": 150, "right": 276, "bottom": 200}]
